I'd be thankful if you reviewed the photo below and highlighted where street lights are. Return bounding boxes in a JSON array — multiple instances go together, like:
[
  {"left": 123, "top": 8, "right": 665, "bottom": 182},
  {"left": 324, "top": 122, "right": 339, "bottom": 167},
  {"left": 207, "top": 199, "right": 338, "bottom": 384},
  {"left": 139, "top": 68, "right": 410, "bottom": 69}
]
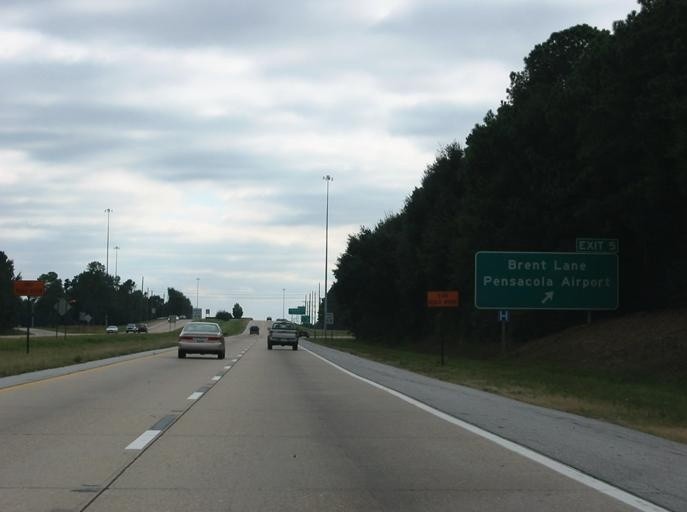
[
  {"left": 282, "top": 288, "right": 287, "bottom": 319},
  {"left": 114, "top": 246, "right": 120, "bottom": 276},
  {"left": 195, "top": 277, "right": 200, "bottom": 307},
  {"left": 104, "top": 208, "right": 114, "bottom": 273},
  {"left": 322, "top": 174, "right": 334, "bottom": 336}
]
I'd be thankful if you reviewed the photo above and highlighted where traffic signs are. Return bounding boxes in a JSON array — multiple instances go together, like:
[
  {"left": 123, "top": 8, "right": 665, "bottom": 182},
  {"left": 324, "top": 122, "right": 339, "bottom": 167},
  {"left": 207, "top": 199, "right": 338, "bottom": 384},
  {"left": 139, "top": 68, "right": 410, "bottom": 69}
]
[{"left": 474, "top": 251, "right": 620, "bottom": 312}]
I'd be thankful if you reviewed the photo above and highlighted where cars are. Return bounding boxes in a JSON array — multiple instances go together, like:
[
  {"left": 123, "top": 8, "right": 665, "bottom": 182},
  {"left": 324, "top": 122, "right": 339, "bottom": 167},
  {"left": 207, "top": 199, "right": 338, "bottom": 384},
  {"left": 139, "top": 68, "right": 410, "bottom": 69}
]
[
  {"left": 178, "top": 322, "right": 224, "bottom": 359},
  {"left": 250, "top": 317, "right": 309, "bottom": 349},
  {"left": 126, "top": 323, "right": 148, "bottom": 334},
  {"left": 106, "top": 325, "right": 119, "bottom": 333}
]
